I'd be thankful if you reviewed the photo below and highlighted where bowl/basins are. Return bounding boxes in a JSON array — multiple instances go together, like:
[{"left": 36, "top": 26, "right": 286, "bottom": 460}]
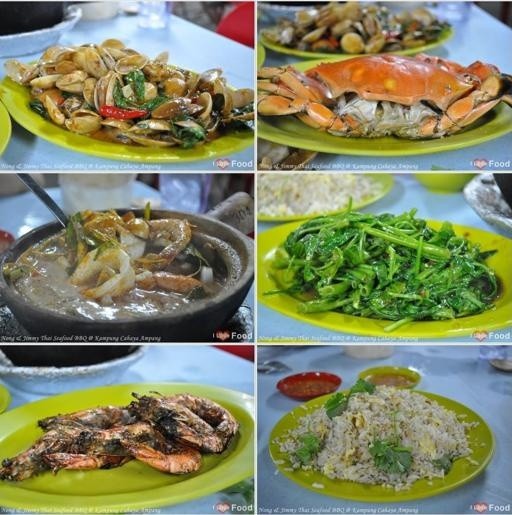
[
  {"left": 345, "top": 344, "right": 399, "bottom": 363},
  {"left": 414, "top": 175, "right": 476, "bottom": 193},
  {"left": 78, "top": 2, "right": 121, "bottom": 22},
  {"left": 0, "top": 189, "right": 257, "bottom": 342},
  {"left": 56, "top": 172, "right": 140, "bottom": 206}
]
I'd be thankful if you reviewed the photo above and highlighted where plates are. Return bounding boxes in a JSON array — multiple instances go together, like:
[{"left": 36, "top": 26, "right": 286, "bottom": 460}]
[
  {"left": 257, "top": 215, "right": 510, "bottom": 341},
  {"left": 1, "top": 61, "right": 255, "bottom": 164},
  {"left": 0, "top": 383, "right": 253, "bottom": 514},
  {"left": 357, "top": 365, "right": 421, "bottom": 388},
  {"left": 260, "top": 24, "right": 453, "bottom": 61},
  {"left": 458, "top": 171, "right": 512, "bottom": 234},
  {"left": 260, "top": 2, "right": 390, "bottom": 20},
  {"left": 278, "top": 372, "right": 344, "bottom": 402},
  {"left": 259, "top": 58, "right": 512, "bottom": 158},
  {"left": 0, "top": 4, "right": 84, "bottom": 58},
  {"left": 257, "top": 40, "right": 266, "bottom": 73},
  {"left": 489, "top": 359, "right": 512, "bottom": 372},
  {"left": 268, "top": 387, "right": 497, "bottom": 503},
  {"left": 0, "top": 345, "right": 151, "bottom": 395},
  {"left": 0, "top": 382, "right": 12, "bottom": 413},
  {"left": 257, "top": 172, "right": 394, "bottom": 222},
  {"left": 1, "top": 101, "right": 11, "bottom": 155}
]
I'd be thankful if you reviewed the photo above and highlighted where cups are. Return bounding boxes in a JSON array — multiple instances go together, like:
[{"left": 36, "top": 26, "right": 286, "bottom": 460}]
[
  {"left": 157, "top": 174, "right": 208, "bottom": 212},
  {"left": 438, "top": 2, "right": 473, "bottom": 25},
  {"left": 136, "top": 2, "right": 171, "bottom": 31}
]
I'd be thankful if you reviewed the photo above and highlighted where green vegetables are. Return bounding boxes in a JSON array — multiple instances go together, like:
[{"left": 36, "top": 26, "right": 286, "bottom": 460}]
[{"left": 262, "top": 197, "right": 499, "bottom": 333}]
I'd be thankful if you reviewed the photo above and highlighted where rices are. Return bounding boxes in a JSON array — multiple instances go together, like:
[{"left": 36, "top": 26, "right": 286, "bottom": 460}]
[
  {"left": 257, "top": 173, "right": 384, "bottom": 215},
  {"left": 274, "top": 385, "right": 485, "bottom": 491}
]
